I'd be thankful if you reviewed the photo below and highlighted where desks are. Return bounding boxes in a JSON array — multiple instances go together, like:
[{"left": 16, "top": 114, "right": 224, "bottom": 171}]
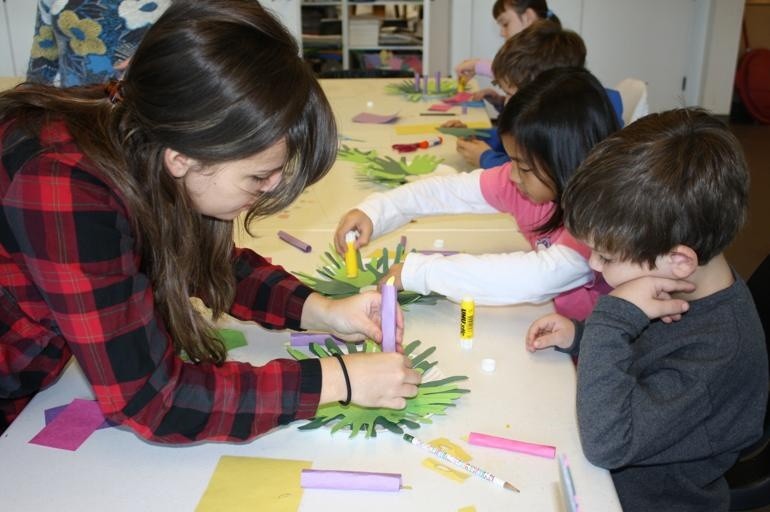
[{"left": 2, "top": 72, "right": 619, "bottom": 511}]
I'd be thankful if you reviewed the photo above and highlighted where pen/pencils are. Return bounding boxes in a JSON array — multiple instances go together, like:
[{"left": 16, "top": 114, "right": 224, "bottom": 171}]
[
  {"left": 421, "top": 114, "right": 456, "bottom": 116},
  {"left": 403, "top": 434, "right": 521, "bottom": 493}
]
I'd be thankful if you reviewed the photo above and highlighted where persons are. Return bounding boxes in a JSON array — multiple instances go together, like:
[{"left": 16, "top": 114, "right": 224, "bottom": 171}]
[
  {"left": 1, "top": 1, "right": 421, "bottom": 444},
  {"left": 439, "top": 20, "right": 624, "bottom": 169},
  {"left": 457, "top": 0, "right": 562, "bottom": 85},
  {"left": 334, "top": 66, "right": 622, "bottom": 321},
  {"left": 527, "top": 107, "right": 770, "bottom": 512}
]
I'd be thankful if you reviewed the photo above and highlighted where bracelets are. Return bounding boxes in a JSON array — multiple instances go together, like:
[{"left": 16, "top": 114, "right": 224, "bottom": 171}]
[{"left": 332, "top": 353, "right": 351, "bottom": 406}]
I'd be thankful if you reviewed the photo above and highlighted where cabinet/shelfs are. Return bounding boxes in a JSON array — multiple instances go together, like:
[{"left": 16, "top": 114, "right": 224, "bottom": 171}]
[{"left": 304, "top": 1, "right": 451, "bottom": 79}]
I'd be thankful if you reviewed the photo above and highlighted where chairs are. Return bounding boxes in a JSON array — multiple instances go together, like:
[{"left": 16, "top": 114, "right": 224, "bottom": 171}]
[
  {"left": 621, "top": 74, "right": 649, "bottom": 129},
  {"left": 727, "top": 252, "right": 767, "bottom": 512}
]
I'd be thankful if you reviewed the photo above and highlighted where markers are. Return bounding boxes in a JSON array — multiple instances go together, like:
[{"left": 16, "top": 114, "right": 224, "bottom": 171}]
[{"left": 420, "top": 137, "right": 442, "bottom": 148}]
